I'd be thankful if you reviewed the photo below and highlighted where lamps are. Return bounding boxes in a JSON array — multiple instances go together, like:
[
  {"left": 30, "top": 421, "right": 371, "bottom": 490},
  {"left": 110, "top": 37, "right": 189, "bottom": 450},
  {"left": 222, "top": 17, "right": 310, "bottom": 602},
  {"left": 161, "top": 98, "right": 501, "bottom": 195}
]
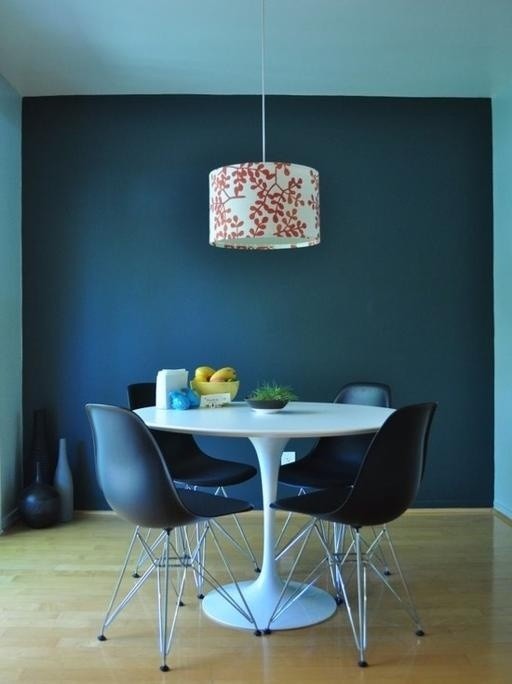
[{"left": 207, "top": 0, "right": 325, "bottom": 253}]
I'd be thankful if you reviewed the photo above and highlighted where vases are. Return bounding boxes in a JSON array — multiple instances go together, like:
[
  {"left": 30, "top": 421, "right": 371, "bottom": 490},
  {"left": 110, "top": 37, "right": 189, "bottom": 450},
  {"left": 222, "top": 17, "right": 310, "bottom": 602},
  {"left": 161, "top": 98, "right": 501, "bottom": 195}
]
[{"left": 17, "top": 407, "right": 76, "bottom": 528}]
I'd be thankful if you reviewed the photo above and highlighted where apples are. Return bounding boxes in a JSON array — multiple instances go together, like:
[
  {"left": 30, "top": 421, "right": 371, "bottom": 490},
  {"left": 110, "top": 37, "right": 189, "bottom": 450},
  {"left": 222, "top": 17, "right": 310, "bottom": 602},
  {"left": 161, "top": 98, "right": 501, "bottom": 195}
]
[
  {"left": 195, "top": 367, "right": 215, "bottom": 382},
  {"left": 209, "top": 367, "right": 237, "bottom": 382}
]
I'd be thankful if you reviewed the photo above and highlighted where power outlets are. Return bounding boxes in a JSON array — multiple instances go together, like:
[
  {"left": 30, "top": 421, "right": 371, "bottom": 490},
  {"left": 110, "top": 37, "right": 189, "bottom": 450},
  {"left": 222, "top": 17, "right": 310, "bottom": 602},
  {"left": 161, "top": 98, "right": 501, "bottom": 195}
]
[{"left": 281, "top": 450, "right": 296, "bottom": 465}]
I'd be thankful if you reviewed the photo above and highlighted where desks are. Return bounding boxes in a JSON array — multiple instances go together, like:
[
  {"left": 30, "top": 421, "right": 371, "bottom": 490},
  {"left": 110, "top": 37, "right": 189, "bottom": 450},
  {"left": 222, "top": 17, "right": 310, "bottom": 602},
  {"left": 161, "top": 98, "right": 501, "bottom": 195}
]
[{"left": 131, "top": 401, "right": 396, "bottom": 632}]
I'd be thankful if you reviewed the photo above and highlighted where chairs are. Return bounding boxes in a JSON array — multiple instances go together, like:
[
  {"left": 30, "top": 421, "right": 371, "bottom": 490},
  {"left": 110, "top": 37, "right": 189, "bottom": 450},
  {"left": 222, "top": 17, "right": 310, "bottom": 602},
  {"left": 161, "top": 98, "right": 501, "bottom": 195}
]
[
  {"left": 276, "top": 382, "right": 390, "bottom": 605},
  {"left": 128, "top": 382, "right": 260, "bottom": 608},
  {"left": 84, "top": 403, "right": 262, "bottom": 672},
  {"left": 271, "top": 402, "right": 438, "bottom": 670}
]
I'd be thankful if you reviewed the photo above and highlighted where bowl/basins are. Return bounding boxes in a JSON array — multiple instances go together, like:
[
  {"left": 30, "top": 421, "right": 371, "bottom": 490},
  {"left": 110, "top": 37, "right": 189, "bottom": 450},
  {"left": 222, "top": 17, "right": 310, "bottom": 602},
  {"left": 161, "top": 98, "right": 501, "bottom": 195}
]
[
  {"left": 243, "top": 396, "right": 290, "bottom": 415},
  {"left": 189, "top": 380, "right": 240, "bottom": 403}
]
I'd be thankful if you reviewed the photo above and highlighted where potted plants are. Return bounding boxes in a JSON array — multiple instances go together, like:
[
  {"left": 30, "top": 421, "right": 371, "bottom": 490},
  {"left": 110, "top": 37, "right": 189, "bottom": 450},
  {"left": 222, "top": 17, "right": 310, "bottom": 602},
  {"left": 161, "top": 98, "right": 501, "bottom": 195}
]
[{"left": 245, "top": 382, "right": 298, "bottom": 414}]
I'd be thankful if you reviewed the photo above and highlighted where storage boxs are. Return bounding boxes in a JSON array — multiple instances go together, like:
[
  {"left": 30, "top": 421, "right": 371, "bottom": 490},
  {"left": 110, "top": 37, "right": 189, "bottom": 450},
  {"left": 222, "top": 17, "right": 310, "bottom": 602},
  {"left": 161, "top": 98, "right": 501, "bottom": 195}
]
[{"left": 155, "top": 374, "right": 187, "bottom": 410}]
